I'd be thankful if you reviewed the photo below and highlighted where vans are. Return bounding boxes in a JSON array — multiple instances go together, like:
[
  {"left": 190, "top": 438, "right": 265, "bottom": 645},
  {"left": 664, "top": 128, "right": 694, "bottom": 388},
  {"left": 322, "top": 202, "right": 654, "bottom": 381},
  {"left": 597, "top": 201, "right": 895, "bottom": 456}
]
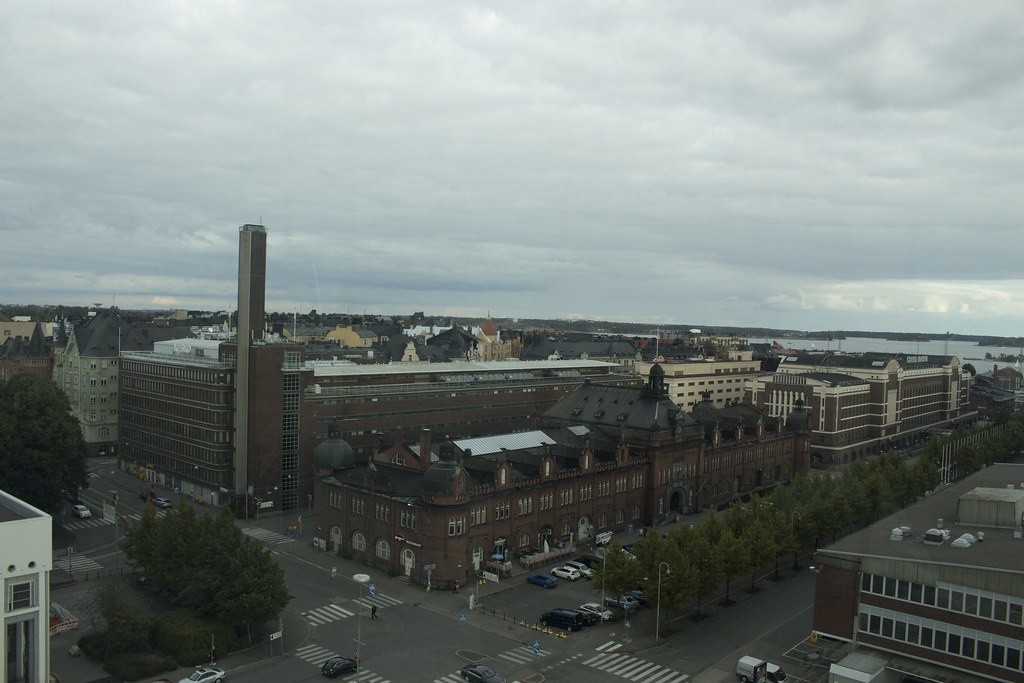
[
  {"left": 562, "top": 554, "right": 603, "bottom": 579},
  {"left": 540, "top": 608, "right": 596, "bottom": 632}
]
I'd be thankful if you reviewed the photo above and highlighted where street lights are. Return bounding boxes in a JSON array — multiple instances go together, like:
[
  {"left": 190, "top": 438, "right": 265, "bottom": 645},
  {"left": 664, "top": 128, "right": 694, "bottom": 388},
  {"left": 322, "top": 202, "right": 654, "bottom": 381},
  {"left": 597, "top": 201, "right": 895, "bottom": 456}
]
[
  {"left": 353, "top": 573, "right": 376, "bottom": 683},
  {"left": 655, "top": 562, "right": 671, "bottom": 642}
]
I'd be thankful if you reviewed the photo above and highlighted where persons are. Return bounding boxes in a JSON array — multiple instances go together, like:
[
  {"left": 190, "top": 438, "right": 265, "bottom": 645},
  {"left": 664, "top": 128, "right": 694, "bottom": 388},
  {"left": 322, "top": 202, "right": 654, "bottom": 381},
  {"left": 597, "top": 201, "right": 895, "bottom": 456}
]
[
  {"left": 453, "top": 580, "right": 459, "bottom": 594},
  {"left": 371, "top": 604, "right": 378, "bottom": 619}
]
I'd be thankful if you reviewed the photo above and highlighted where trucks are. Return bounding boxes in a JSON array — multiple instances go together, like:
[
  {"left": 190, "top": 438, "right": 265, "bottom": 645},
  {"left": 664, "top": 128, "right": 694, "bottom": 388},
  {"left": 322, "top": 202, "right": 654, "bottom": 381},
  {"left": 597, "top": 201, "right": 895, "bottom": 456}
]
[{"left": 735, "top": 655, "right": 789, "bottom": 683}]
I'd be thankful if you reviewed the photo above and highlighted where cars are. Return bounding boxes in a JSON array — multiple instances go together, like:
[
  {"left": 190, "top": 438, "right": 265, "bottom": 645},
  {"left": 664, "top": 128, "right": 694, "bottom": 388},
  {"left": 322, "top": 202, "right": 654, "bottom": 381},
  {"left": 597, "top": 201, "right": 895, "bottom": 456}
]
[
  {"left": 178, "top": 667, "right": 226, "bottom": 683},
  {"left": 604, "top": 590, "right": 645, "bottom": 610},
  {"left": 580, "top": 602, "right": 614, "bottom": 621},
  {"left": 72, "top": 504, "right": 91, "bottom": 518},
  {"left": 152, "top": 496, "right": 171, "bottom": 507},
  {"left": 321, "top": 656, "right": 357, "bottom": 678},
  {"left": 461, "top": 664, "right": 506, "bottom": 683}
]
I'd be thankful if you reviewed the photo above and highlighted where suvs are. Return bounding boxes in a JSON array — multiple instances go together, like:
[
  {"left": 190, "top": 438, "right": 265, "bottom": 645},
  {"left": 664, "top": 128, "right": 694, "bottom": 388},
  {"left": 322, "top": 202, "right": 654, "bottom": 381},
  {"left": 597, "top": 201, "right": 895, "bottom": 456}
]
[
  {"left": 527, "top": 573, "right": 558, "bottom": 589},
  {"left": 550, "top": 566, "right": 581, "bottom": 581}
]
